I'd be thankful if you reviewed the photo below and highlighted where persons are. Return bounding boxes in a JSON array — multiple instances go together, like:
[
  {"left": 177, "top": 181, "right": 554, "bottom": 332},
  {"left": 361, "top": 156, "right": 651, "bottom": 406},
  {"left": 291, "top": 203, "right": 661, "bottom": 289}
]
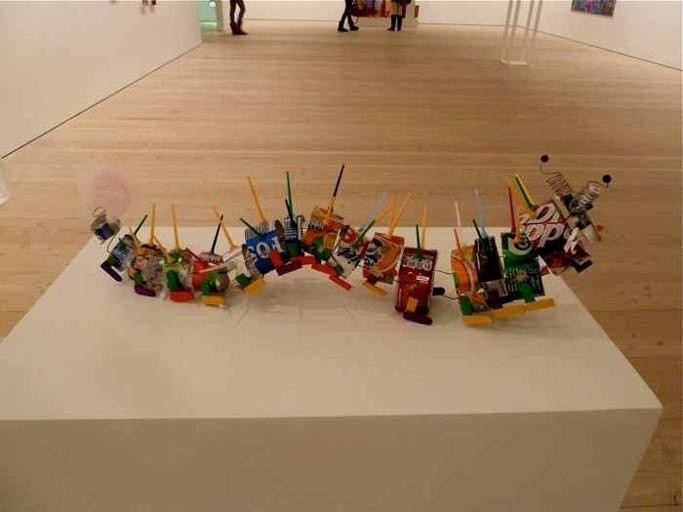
[
  {"left": 228, "top": 1, "right": 247, "bottom": 36},
  {"left": 337, "top": 0, "right": 359, "bottom": 32},
  {"left": 384, "top": 0, "right": 411, "bottom": 33}
]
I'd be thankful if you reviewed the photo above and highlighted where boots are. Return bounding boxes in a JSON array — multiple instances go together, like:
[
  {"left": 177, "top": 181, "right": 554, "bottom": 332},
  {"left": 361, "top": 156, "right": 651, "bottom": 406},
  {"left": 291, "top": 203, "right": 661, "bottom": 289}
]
[
  {"left": 229, "top": 20, "right": 247, "bottom": 36},
  {"left": 337, "top": 22, "right": 348, "bottom": 32},
  {"left": 348, "top": 21, "right": 359, "bottom": 31}
]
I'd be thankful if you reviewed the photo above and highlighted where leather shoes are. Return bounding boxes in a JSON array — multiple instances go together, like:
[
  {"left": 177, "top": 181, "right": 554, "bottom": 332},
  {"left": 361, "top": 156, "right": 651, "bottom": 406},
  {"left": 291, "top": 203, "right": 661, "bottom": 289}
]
[{"left": 387, "top": 27, "right": 402, "bottom": 31}]
